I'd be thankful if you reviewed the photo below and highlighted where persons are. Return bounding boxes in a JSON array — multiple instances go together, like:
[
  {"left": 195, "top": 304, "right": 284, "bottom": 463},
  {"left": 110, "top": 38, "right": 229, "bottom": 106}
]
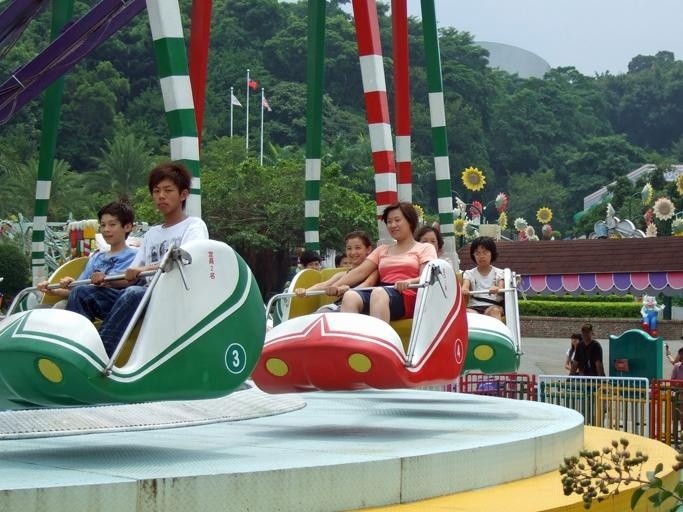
[
  {"left": 325, "top": 202, "right": 438, "bottom": 323},
  {"left": 668, "top": 346, "right": 683, "bottom": 440},
  {"left": 294, "top": 232, "right": 373, "bottom": 312},
  {"left": 461, "top": 237, "right": 506, "bottom": 321},
  {"left": 32, "top": 201, "right": 137, "bottom": 309},
  {"left": 301, "top": 250, "right": 321, "bottom": 271},
  {"left": 335, "top": 254, "right": 350, "bottom": 268},
  {"left": 664, "top": 336, "right": 683, "bottom": 366},
  {"left": 570, "top": 324, "right": 605, "bottom": 376},
  {"left": 565, "top": 334, "right": 581, "bottom": 375},
  {"left": 414, "top": 226, "right": 454, "bottom": 268},
  {"left": 65, "top": 164, "right": 209, "bottom": 359}
]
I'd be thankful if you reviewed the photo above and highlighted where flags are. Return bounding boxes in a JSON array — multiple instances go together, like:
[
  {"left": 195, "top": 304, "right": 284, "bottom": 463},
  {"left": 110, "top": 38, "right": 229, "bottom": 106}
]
[
  {"left": 249, "top": 79, "right": 264, "bottom": 92},
  {"left": 263, "top": 96, "right": 273, "bottom": 112},
  {"left": 231, "top": 95, "right": 243, "bottom": 109}
]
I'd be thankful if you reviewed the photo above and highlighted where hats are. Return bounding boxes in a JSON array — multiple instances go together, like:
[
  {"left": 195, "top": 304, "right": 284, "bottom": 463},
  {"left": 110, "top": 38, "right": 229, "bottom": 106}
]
[{"left": 582, "top": 325, "right": 593, "bottom": 332}]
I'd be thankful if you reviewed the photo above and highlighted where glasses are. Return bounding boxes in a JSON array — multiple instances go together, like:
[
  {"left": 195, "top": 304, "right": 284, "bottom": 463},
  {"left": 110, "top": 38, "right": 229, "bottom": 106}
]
[{"left": 473, "top": 251, "right": 492, "bottom": 257}]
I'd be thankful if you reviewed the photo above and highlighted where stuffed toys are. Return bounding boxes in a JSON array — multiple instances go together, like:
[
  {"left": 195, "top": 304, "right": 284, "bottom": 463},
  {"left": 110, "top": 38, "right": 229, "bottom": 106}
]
[{"left": 639, "top": 293, "right": 667, "bottom": 337}]
